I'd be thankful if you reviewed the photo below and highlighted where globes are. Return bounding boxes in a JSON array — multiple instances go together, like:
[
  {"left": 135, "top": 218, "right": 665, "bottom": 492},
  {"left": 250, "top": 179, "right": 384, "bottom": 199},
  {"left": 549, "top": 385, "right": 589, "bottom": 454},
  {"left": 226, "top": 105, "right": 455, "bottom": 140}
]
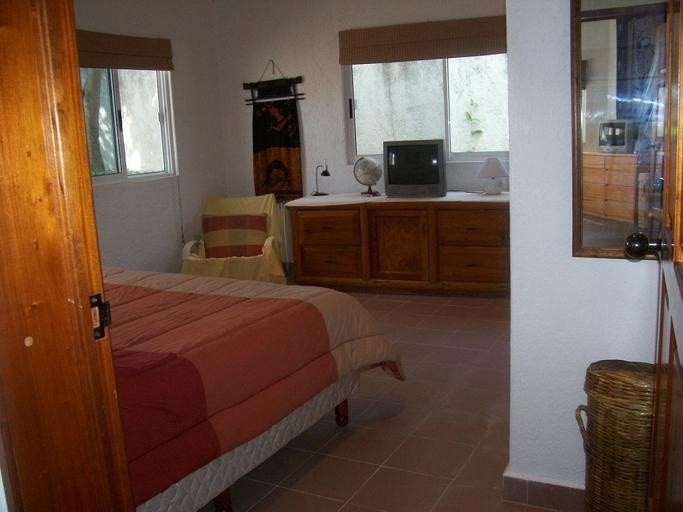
[{"left": 353, "top": 157, "right": 383, "bottom": 196}]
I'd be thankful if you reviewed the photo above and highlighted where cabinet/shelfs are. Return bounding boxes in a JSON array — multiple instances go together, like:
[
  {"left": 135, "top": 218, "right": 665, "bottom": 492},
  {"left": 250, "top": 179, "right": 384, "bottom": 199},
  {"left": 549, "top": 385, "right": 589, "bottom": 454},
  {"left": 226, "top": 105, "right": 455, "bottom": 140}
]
[
  {"left": 582, "top": 149, "right": 651, "bottom": 224},
  {"left": 284, "top": 191, "right": 510, "bottom": 298}
]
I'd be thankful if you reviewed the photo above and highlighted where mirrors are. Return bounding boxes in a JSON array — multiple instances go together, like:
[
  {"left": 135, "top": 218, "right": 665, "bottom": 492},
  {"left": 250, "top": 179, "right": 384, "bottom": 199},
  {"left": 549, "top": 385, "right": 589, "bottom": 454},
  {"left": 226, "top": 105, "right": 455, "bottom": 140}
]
[{"left": 572, "top": 0, "right": 679, "bottom": 263}]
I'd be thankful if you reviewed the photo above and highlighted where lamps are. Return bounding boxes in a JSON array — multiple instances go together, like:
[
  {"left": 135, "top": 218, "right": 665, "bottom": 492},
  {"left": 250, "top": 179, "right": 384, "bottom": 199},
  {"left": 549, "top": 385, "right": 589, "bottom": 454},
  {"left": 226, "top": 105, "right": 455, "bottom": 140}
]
[
  {"left": 311, "top": 165, "right": 330, "bottom": 196},
  {"left": 473, "top": 158, "right": 509, "bottom": 194}
]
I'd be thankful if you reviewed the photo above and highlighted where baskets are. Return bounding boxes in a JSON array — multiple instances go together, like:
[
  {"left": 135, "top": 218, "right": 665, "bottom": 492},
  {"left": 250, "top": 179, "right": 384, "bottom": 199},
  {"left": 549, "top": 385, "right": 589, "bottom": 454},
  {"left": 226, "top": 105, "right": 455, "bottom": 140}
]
[{"left": 575, "top": 359, "right": 655, "bottom": 512}]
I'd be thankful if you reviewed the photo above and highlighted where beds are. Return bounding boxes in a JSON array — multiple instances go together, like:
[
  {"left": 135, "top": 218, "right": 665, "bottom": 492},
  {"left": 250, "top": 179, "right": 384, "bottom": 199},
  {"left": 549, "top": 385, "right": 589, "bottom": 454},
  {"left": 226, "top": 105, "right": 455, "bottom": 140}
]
[{"left": 100, "top": 268, "right": 405, "bottom": 512}]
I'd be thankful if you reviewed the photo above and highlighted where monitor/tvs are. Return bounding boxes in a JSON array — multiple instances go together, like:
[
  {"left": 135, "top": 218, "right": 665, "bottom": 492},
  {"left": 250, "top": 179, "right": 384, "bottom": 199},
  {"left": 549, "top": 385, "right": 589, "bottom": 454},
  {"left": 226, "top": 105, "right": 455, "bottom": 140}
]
[
  {"left": 383, "top": 139, "right": 447, "bottom": 198},
  {"left": 598, "top": 119, "right": 634, "bottom": 153}
]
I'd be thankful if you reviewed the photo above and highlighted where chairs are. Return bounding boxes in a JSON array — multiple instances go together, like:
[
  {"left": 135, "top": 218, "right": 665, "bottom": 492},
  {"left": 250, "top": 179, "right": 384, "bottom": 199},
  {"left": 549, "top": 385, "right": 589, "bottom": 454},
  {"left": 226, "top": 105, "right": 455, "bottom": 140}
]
[{"left": 180, "top": 193, "right": 286, "bottom": 284}]
